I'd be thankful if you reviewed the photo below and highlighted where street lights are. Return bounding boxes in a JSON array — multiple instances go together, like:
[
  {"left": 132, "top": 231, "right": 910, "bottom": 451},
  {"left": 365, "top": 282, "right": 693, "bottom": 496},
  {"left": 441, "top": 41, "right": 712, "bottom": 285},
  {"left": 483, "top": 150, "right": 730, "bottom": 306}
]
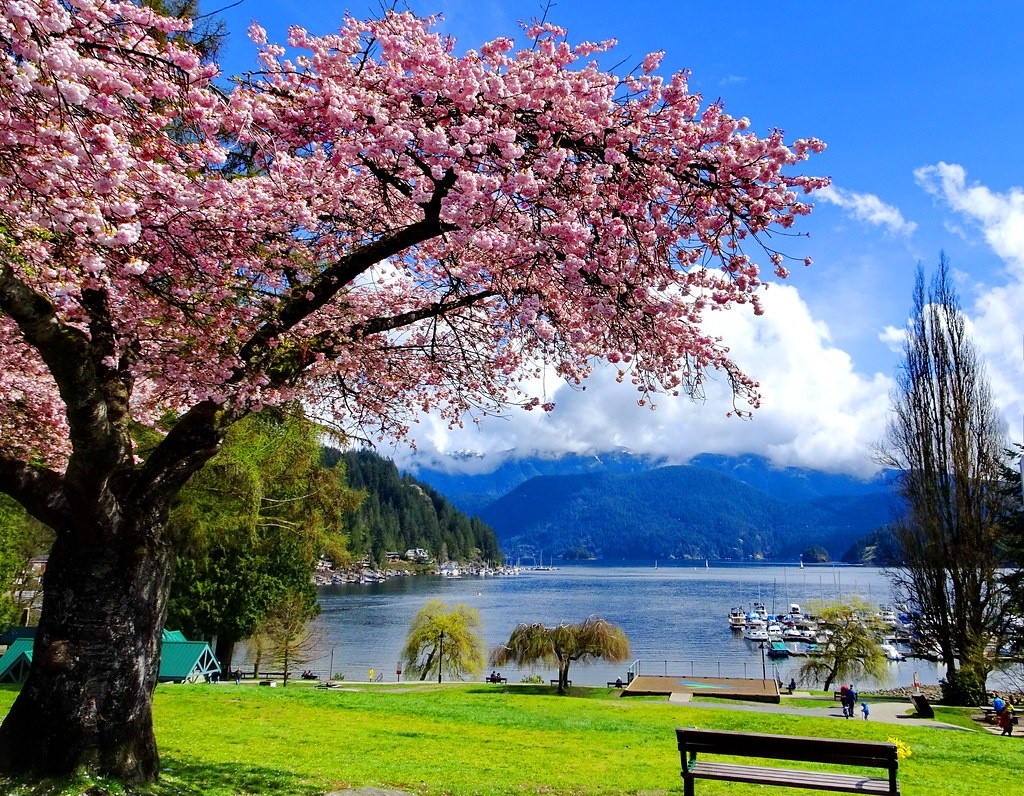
[
  {"left": 439, "top": 630, "right": 446, "bottom": 683},
  {"left": 757, "top": 642, "right": 766, "bottom": 679}
]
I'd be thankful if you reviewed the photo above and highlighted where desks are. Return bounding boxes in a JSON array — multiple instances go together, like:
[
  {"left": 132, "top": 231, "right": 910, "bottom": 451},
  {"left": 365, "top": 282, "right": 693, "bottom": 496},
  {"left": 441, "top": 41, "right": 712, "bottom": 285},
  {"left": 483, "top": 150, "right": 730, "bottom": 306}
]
[{"left": 979, "top": 706, "right": 1024, "bottom": 722}]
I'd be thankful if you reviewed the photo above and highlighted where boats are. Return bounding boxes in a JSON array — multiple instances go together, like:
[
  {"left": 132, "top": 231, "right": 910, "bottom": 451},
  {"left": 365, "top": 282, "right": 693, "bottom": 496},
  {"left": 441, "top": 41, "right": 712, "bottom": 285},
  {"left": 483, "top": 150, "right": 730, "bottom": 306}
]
[{"left": 446, "top": 575, "right": 462, "bottom": 579}]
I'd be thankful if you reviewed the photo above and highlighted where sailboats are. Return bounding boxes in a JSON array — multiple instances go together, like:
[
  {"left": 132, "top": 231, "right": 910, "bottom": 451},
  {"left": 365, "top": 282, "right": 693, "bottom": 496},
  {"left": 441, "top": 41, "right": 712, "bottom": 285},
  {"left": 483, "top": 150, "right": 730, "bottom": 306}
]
[
  {"left": 799, "top": 561, "right": 804, "bottom": 569},
  {"left": 479, "top": 550, "right": 555, "bottom": 575},
  {"left": 727, "top": 564, "right": 944, "bottom": 662}
]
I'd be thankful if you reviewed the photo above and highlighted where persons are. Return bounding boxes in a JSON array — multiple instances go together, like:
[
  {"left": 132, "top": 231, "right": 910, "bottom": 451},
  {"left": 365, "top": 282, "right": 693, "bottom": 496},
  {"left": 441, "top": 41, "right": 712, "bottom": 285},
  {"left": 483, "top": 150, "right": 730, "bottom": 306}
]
[
  {"left": 786, "top": 678, "right": 796, "bottom": 690},
  {"left": 369, "top": 667, "right": 374, "bottom": 682},
  {"left": 615, "top": 676, "right": 623, "bottom": 689},
  {"left": 234, "top": 667, "right": 243, "bottom": 685},
  {"left": 840, "top": 684, "right": 870, "bottom": 721},
  {"left": 490, "top": 671, "right": 501, "bottom": 684},
  {"left": 993, "top": 695, "right": 1015, "bottom": 737},
  {"left": 301, "top": 670, "right": 314, "bottom": 678}
]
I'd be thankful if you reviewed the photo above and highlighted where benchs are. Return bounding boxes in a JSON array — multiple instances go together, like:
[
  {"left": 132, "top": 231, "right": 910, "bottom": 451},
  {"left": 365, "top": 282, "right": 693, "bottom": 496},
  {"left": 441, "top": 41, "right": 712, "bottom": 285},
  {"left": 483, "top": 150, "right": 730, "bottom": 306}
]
[
  {"left": 607, "top": 682, "right": 628, "bottom": 687},
  {"left": 675, "top": 726, "right": 901, "bottom": 796},
  {"left": 982, "top": 710, "right": 1021, "bottom": 724},
  {"left": 834, "top": 691, "right": 857, "bottom": 702},
  {"left": 486, "top": 678, "right": 507, "bottom": 684},
  {"left": 301, "top": 674, "right": 318, "bottom": 679},
  {"left": 551, "top": 679, "right": 572, "bottom": 686}
]
